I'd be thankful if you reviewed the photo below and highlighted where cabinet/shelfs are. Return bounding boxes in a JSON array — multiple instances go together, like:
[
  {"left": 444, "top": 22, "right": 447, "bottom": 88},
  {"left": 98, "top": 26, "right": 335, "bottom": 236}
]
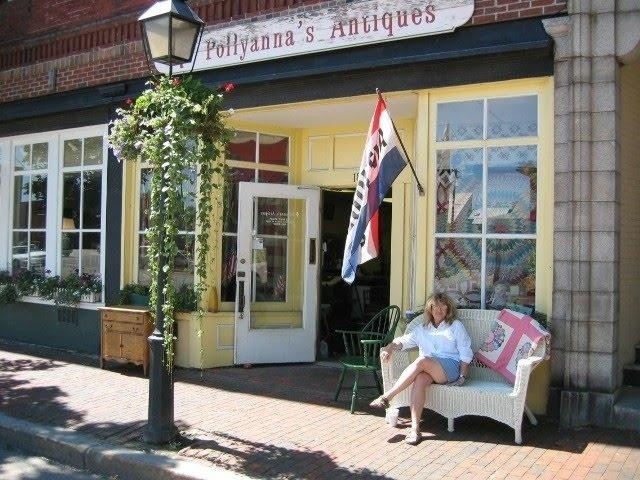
[{"left": 97, "top": 304, "right": 153, "bottom": 378}]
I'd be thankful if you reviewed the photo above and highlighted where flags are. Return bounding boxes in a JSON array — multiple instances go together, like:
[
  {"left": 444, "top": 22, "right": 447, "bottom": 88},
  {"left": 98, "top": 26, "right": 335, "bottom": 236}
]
[{"left": 341, "top": 98, "right": 408, "bottom": 287}]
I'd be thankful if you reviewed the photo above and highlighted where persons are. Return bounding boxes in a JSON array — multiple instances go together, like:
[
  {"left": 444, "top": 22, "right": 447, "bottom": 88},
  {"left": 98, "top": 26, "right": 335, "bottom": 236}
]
[{"left": 370, "top": 291, "right": 473, "bottom": 446}]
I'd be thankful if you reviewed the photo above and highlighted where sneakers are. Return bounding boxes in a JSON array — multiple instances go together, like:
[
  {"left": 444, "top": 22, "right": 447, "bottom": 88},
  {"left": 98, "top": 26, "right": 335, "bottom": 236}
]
[
  {"left": 405, "top": 432, "right": 422, "bottom": 444},
  {"left": 370, "top": 396, "right": 390, "bottom": 410}
]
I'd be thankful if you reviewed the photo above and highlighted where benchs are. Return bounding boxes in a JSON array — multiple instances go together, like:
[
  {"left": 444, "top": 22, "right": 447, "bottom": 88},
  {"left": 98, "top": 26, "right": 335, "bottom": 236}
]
[{"left": 377, "top": 306, "right": 552, "bottom": 446}]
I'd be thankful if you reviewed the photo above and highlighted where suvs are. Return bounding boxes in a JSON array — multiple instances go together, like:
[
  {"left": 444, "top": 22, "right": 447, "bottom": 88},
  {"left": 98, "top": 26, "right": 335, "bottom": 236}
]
[{"left": 140, "top": 245, "right": 194, "bottom": 273}]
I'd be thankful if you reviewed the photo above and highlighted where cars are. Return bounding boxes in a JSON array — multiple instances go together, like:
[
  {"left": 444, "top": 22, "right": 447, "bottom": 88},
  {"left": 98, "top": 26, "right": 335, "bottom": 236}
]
[
  {"left": 30, "top": 243, "right": 45, "bottom": 267},
  {"left": 12, "top": 245, "right": 28, "bottom": 269}
]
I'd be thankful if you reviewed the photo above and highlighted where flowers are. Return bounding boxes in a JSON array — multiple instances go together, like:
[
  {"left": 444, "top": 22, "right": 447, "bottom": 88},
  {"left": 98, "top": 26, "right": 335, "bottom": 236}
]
[
  {"left": 105, "top": 69, "right": 238, "bottom": 386},
  {"left": 0, "top": 267, "right": 102, "bottom": 306}
]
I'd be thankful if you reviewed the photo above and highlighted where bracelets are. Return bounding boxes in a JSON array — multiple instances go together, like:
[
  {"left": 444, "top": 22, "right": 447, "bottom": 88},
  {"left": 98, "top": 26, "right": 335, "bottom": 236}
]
[{"left": 458, "top": 375, "right": 465, "bottom": 378}]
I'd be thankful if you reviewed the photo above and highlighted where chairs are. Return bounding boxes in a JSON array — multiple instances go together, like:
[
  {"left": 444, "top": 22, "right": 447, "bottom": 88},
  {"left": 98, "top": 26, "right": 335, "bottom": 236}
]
[{"left": 332, "top": 304, "right": 401, "bottom": 414}]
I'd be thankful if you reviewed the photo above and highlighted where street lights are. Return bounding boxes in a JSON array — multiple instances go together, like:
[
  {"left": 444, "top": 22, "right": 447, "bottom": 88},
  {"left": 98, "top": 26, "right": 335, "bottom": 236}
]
[{"left": 133, "top": 1, "right": 207, "bottom": 445}]
